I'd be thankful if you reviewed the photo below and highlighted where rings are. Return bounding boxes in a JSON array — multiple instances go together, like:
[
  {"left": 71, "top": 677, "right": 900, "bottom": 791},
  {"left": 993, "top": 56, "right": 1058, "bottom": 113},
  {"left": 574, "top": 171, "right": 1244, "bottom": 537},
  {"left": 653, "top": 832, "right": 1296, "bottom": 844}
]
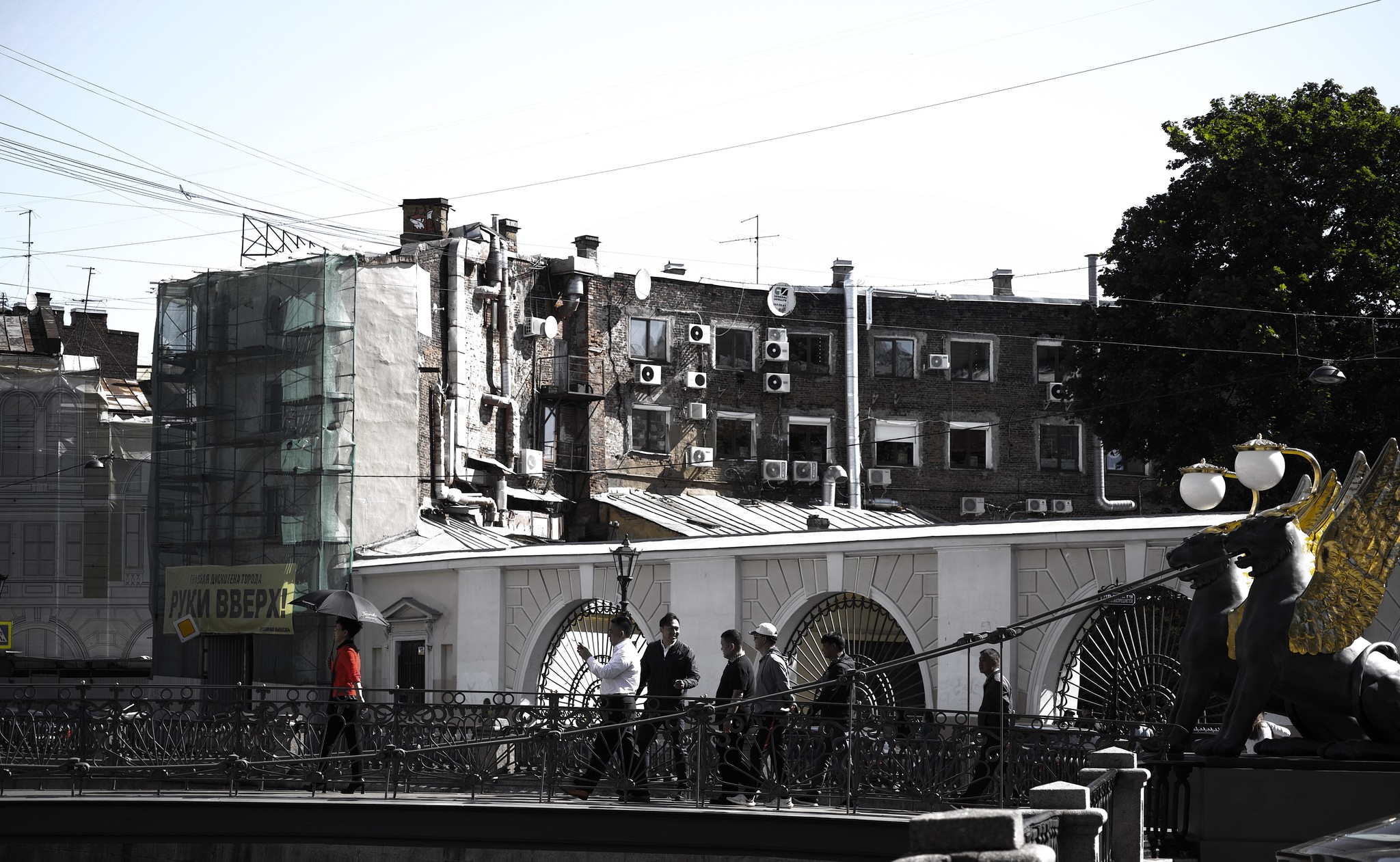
[{"left": 578, "top": 648, "right": 581, "bottom": 651}]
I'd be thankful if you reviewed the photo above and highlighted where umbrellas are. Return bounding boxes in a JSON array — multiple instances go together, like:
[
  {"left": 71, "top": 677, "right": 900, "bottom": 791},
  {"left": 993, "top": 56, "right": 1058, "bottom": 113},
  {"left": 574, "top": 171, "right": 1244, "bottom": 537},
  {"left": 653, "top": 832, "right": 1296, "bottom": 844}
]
[{"left": 287, "top": 582, "right": 394, "bottom": 668}]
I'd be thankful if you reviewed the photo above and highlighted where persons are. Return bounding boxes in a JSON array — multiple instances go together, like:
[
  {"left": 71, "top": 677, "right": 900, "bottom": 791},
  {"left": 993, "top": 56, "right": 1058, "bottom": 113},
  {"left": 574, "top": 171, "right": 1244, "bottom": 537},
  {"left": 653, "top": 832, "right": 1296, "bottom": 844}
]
[
  {"left": 793, "top": 631, "right": 859, "bottom": 809},
  {"left": 726, "top": 622, "right": 797, "bottom": 808},
  {"left": 946, "top": 648, "right": 1019, "bottom": 809},
  {"left": 1162, "top": 720, "right": 1167, "bottom": 724},
  {"left": 1061, "top": 709, "right": 1075, "bottom": 726},
  {"left": 559, "top": 617, "right": 650, "bottom": 802},
  {"left": 710, "top": 629, "right": 756, "bottom": 804},
  {"left": 1075, "top": 703, "right": 1107, "bottom": 734},
  {"left": 1245, "top": 712, "right": 1291, "bottom": 753},
  {"left": 1130, "top": 711, "right": 1154, "bottom": 751},
  {"left": 302, "top": 616, "right": 365, "bottom": 794},
  {"left": 635, "top": 611, "right": 700, "bottom": 802}
]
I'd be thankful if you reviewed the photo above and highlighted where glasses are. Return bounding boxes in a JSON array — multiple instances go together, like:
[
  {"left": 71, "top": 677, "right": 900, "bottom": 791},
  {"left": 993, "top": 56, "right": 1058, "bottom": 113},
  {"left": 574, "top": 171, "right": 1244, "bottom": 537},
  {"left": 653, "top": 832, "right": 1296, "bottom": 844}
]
[
  {"left": 754, "top": 635, "right": 762, "bottom": 640},
  {"left": 334, "top": 628, "right": 341, "bottom": 631}
]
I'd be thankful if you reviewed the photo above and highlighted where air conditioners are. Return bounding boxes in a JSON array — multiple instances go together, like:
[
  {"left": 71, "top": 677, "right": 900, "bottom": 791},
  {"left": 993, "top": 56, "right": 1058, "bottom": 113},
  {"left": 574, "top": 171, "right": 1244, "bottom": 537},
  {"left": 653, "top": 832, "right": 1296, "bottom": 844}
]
[
  {"left": 683, "top": 371, "right": 706, "bottom": 389},
  {"left": 928, "top": 354, "right": 948, "bottom": 369},
  {"left": 684, "top": 324, "right": 710, "bottom": 344},
  {"left": 761, "top": 460, "right": 787, "bottom": 479},
  {"left": 762, "top": 341, "right": 789, "bottom": 361},
  {"left": 687, "top": 446, "right": 713, "bottom": 467},
  {"left": 960, "top": 497, "right": 984, "bottom": 514},
  {"left": 766, "top": 327, "right": 786, "bottom": 342},
  {"left": 1051, "top": 499, "right": 1071, "bottom": 513},
  {"left": 635, "top": 364, "right": 661, "bottom": 386},
  {"left": 867, "top": 468, "right": 890, "bottom": 484},
  {"left": 792, "top": 461, "right": 818, "bottom": 481},
  {"left": 1026, "top": 499, "right": 1047, "bottom": 512},
  {"left": 1047, "top": 382, "right": 1073, "bottom": 403},
  {"left": 763, "top": 373, "right": 790, "bottom": 393},
  {"left": 527, "top": 318, "right": 545, "bottom": 335},
  {"left": 687, "top": 403, "right": 707, "bottom": 421},
  {"left": 518, "top": 447, "right": 543, "bottom": 477}
]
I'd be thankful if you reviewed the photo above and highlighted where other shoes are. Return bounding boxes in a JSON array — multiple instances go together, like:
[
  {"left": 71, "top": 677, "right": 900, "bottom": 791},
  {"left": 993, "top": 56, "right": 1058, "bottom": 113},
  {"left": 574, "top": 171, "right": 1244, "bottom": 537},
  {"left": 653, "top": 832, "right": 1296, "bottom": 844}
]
[
  {"left": 618, "top": 793, "right": 650, "bottom": 803},
  {"left": 559, "top": 785, "right": 590, "bottom": 801}
]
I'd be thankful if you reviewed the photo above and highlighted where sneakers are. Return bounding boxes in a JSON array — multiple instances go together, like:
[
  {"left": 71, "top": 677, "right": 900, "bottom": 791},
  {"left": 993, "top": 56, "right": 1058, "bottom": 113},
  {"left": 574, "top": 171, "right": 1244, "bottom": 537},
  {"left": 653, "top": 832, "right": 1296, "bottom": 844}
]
[
  {"left": 726, "top": 793, "right": 756, "bottom": 807},
  {"left": 836, "top": 798, "right": 859, "bottom": 810},
  {"left": 793, "top": 794, "right": 819, "bottom": 807},
  {"left": 763, "top": 796, "right": 794, "bottom": 809},
  {"left": 947, "top": 799, "right": 975, "bottom": 810},
  {"left": 675, "top": 791, "right": 688, "bottom": 801},
  {"left": 709, "top": 793, "right": 737, "bottom": 808}
]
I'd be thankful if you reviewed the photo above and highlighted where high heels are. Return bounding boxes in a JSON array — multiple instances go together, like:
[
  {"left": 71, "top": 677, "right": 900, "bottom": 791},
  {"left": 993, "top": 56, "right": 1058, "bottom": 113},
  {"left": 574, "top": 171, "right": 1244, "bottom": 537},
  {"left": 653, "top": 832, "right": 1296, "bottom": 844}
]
[
  {"left": 307, "top": 781, "right": 327, "bottom": 793},
  {"left": 341, "top": 778, "right": 366, "bottom": 794}
]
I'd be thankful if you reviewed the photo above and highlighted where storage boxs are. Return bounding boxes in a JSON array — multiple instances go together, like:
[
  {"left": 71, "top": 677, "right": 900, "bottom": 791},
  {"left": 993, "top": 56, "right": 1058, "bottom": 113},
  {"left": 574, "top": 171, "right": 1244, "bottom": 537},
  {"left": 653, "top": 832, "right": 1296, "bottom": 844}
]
[
  {"left": 567, "top": 383, "right": 586, "bottom": 392},
  {"left": 541, "top": 385, "right": 559, "bottom": 393}
]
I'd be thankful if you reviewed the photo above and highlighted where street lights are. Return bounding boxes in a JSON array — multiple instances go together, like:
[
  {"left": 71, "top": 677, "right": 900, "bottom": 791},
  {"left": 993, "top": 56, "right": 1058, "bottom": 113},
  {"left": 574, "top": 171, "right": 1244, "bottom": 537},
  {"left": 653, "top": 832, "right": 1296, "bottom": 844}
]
[{"left": 607, "top": 534, "right": 644, "bottom": 620}]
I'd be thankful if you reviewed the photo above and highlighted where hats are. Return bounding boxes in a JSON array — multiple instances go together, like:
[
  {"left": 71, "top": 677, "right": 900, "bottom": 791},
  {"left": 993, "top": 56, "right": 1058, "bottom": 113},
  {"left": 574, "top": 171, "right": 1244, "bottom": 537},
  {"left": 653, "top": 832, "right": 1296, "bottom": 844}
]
[{"left": 748, "top": 623, "right": 778, "bottom": 637}]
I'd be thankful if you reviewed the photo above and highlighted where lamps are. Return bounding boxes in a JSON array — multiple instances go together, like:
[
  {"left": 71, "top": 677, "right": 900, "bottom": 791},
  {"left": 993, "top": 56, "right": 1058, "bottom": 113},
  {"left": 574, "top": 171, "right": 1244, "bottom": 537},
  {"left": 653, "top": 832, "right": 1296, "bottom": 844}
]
[
  {"left": 83, "top": 456, "right": 106, "bottom": 469},
  {"left": 1308, "top": 359, "right": 1347, "bottom": 384},
  {"left": 1179, "top": 433, "right": 1322, "bottom": 534}
]
